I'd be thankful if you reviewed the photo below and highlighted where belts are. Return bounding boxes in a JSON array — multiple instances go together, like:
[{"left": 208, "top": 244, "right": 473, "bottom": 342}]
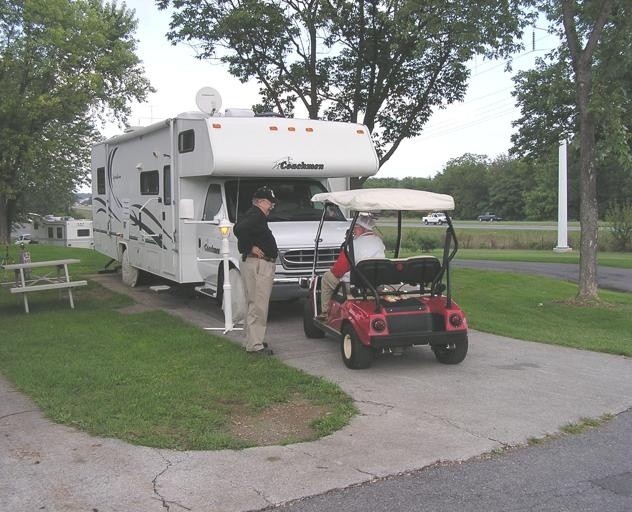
[{"left": 245, "top": 252, "right": 277, "bottom": 263}]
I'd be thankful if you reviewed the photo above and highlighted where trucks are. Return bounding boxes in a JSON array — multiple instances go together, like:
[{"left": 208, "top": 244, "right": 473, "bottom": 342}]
[
  {"left": 34, "top": 210, "right": 94, "bottom": 251},
  {"left": 89, "top": 82, "right": 384, "bottom": 316}
]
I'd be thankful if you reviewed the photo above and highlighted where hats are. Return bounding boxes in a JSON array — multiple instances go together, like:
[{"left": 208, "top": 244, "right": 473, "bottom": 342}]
[
  {"left": 253, "top": 186, "right": 278, "bottom": 203},
  {"left": 346, "top": 214, "right": 377, "bottom": 231}
]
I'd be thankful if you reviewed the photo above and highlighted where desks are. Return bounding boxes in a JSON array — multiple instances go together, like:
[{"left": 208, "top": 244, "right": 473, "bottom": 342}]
[{"left": 0, "top": 258, "right": 81, "bottom": 313}]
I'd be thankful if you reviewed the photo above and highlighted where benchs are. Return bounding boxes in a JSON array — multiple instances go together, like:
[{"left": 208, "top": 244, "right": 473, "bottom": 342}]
[
  {"left": 334, "top": 254, "right": 443, "bottom": 301},
  {"left": 1, "top": 275, "right": 73, "bottom": 307},
  {"left": 9, "top": 279, "right": 88, "bottom": 314}
]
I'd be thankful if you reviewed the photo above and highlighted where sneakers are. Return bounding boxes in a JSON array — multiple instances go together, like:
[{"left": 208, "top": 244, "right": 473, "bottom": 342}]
[
  {"left": 316, "top": 314, "right": 329, "bottom": 321},
  {"left": 242, "top": 342, "right": 267, "bottom": 350},
  {"left": 247, "top": 348, "right": 273, "bottom": 357}
]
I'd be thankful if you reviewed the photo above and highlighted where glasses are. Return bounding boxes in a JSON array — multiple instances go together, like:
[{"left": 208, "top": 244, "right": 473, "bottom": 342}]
[{"left": 258, "top": 199, "right": 276, "bottom": 208}]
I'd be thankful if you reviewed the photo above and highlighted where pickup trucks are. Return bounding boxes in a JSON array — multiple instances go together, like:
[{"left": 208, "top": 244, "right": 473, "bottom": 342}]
[
  {"left": 477, "top": 212, "right": 505, "bottom": 222},
  {"left": 421, "top": 212, "right": 452, "bottom": 225}
]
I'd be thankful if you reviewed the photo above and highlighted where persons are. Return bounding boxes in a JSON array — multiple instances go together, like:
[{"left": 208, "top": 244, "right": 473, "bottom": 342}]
[
  {"left": 315, "top": 214, "right": 386, "bottom": 321},
  {"left": 231, "top": 184, "right": 280, "bottom": 357}
]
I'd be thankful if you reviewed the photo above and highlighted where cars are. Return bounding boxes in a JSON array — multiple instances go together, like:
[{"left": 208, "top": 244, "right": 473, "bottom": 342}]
[{"left": 12, "top": 236, "right": 31, "bottom": 245}]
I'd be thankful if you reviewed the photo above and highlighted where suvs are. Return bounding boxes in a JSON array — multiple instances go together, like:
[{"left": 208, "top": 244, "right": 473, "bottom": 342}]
[{"left": 303, "top": 190, "right": 473, "bottom": 364}]
